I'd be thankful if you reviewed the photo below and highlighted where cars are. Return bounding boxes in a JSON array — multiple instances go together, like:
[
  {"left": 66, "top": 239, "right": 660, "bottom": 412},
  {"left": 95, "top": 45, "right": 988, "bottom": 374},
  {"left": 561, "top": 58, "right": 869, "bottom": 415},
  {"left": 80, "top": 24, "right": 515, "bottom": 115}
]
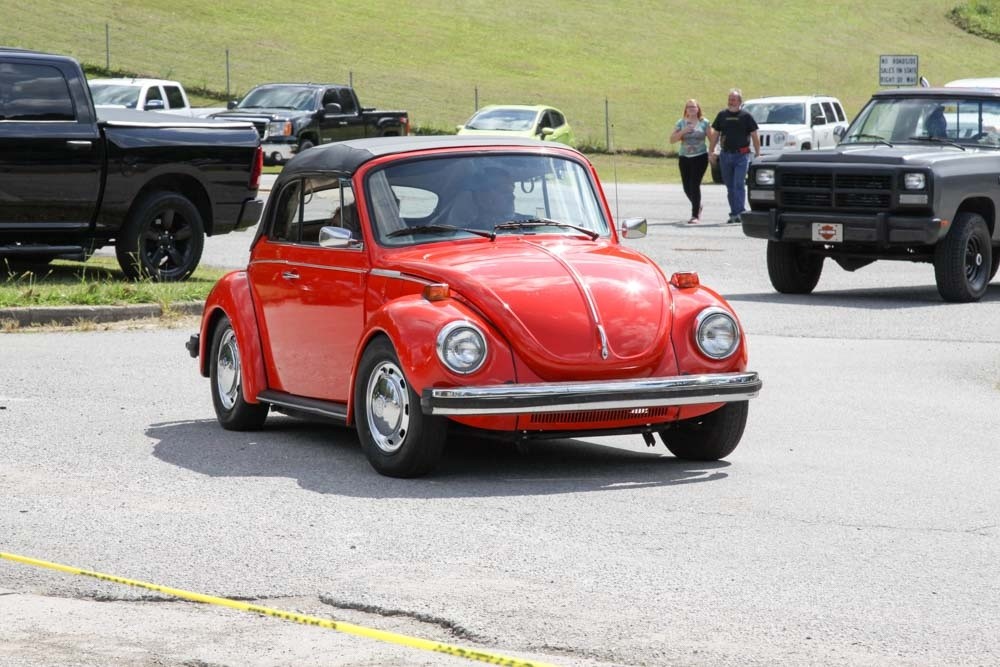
[
  {"left": 183, "top": 132, "right": 764, "bottom": 479},
  {"left": 452, "top": 102, "right": 578, "bottom": 149}
]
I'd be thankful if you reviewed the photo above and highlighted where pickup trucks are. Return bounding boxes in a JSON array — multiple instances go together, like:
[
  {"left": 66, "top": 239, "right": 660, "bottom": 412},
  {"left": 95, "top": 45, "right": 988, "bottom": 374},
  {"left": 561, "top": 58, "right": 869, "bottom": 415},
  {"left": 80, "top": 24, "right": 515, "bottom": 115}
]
[
  {"left": 391, "top": 166, "right": 410, "bottom": 168},
  {"left": 85, "top": 76, "right": 227, "bottom": 120},
  {"left": 0, "top": 48, "right": 265, "bottom": 284},
  {"left": 203, "top": 81, "right": 410, "bottom": 165}
]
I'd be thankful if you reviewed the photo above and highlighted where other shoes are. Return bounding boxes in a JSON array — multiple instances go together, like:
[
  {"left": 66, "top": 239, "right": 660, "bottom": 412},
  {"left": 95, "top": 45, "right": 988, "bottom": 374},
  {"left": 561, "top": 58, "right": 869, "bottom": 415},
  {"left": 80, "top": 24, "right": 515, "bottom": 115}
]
[
  {"left": 729, "top": 213, "right": 738, "bottom": 216},
  {"left": 698, "top": 206, "right": 702, "bottom": 220},
  {"left": 728, "top": 218, "right": 741, "bottom": 223},
  {"left": 689, "top": 217, "right": 703, "bottom": 224}
]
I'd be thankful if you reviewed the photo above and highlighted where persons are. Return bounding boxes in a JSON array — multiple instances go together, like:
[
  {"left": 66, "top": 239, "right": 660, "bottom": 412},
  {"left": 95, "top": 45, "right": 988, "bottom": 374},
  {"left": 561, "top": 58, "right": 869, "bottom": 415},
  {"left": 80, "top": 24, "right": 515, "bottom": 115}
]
[
  {"left": 669, "top": 99, "right": 713, "bottom": 224},
  {"left": 924, "top": 105, "right": 948, "bottom": 140},
  {"left": 330, "top": 183, "right": 400, "bottom": 248},
  {"left": 708, "top": 89, "right": 760, "bottom": 223},
  {"left": 451, "top": 167, "right": 537, "bottom": 238}
]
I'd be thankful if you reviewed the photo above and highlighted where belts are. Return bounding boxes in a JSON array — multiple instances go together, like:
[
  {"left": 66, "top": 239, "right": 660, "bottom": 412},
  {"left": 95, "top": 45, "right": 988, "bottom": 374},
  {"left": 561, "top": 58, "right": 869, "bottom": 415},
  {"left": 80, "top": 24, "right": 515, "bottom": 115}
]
[{"left": 724, "top": 150, "right": 741, "bottom": 154}]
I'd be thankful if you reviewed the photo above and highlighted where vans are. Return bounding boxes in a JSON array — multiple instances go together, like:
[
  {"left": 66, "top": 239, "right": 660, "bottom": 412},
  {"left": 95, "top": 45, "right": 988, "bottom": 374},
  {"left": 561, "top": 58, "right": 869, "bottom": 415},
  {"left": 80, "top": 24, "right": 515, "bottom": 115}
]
[{"left": 705, "top": 95, "right": 851, "bottom": 184}]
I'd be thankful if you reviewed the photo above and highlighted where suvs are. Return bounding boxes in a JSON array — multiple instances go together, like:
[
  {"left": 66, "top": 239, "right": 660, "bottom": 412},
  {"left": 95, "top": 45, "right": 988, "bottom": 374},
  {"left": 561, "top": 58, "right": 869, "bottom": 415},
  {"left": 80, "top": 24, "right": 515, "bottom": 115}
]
[{"left": 739, "top": 75, "right": 1000, "bottom": 305}]
